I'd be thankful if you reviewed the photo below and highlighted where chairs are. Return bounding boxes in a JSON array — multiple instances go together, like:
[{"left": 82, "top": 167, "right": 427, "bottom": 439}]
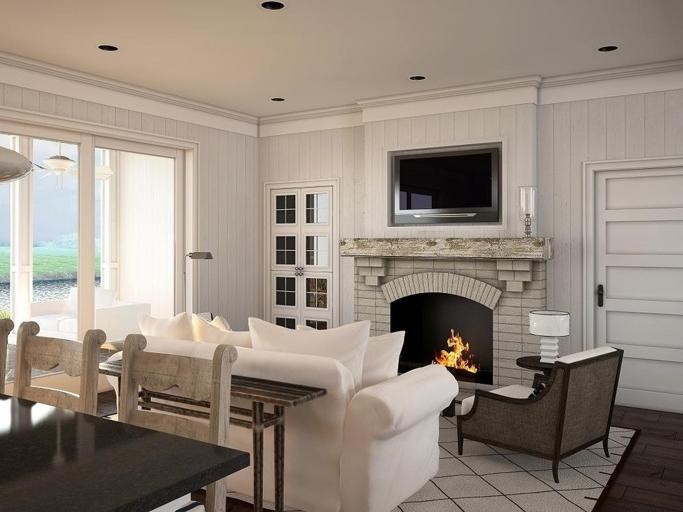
[
  {"left": 32, "top": 300, "right": 151, "bottom": 342},
  {"left": 457, "top": 346, "right": 624, "bottom": 483}
]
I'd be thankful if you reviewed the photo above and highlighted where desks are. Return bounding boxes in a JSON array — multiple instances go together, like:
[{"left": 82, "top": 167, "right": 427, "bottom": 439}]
[{"left": 98, "top": 360, "right": 326, "bottom": 512}]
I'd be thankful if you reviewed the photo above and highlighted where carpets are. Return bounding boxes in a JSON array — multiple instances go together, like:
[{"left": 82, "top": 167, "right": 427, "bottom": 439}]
[{"left": 391, "top": 400, "right": 641, "bottom": 512}]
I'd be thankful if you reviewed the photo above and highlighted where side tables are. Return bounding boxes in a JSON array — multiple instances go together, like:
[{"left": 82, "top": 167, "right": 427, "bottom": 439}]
[{"left": 516, "top": 356, "right": 558, "bottom": 375}]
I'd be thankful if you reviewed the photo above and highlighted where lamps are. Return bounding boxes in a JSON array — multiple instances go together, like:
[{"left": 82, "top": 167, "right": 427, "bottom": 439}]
[
  {"left": 528, "top": 309, "right": 570, "bottom": 363},
  {"left": 518, "top": 186, "right": 536, "bottom": 238},
  {"left": 45, "top": 140, "right": 76, "bottom": 174},
  {"left": 183, "top": 253, "right": 212, "bottom": 269}
]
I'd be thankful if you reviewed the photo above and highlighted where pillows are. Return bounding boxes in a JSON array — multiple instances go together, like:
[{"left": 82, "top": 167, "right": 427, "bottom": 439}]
[
  {"left": 66, "top": 286, "right": 113, "bottom": 312},
  {"left": 137, "top": 313, "right": 407, "bottom": 389}
]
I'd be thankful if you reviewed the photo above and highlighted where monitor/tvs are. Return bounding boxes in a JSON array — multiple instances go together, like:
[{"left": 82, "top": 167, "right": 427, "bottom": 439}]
[{"left": 392, "top": 148, "right": 501, "bottom": 225}]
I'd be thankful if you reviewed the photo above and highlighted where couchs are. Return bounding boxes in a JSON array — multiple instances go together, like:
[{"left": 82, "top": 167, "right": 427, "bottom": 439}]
[{"left": 106, "top": 340, "right": 460, "bottom": 512}]
